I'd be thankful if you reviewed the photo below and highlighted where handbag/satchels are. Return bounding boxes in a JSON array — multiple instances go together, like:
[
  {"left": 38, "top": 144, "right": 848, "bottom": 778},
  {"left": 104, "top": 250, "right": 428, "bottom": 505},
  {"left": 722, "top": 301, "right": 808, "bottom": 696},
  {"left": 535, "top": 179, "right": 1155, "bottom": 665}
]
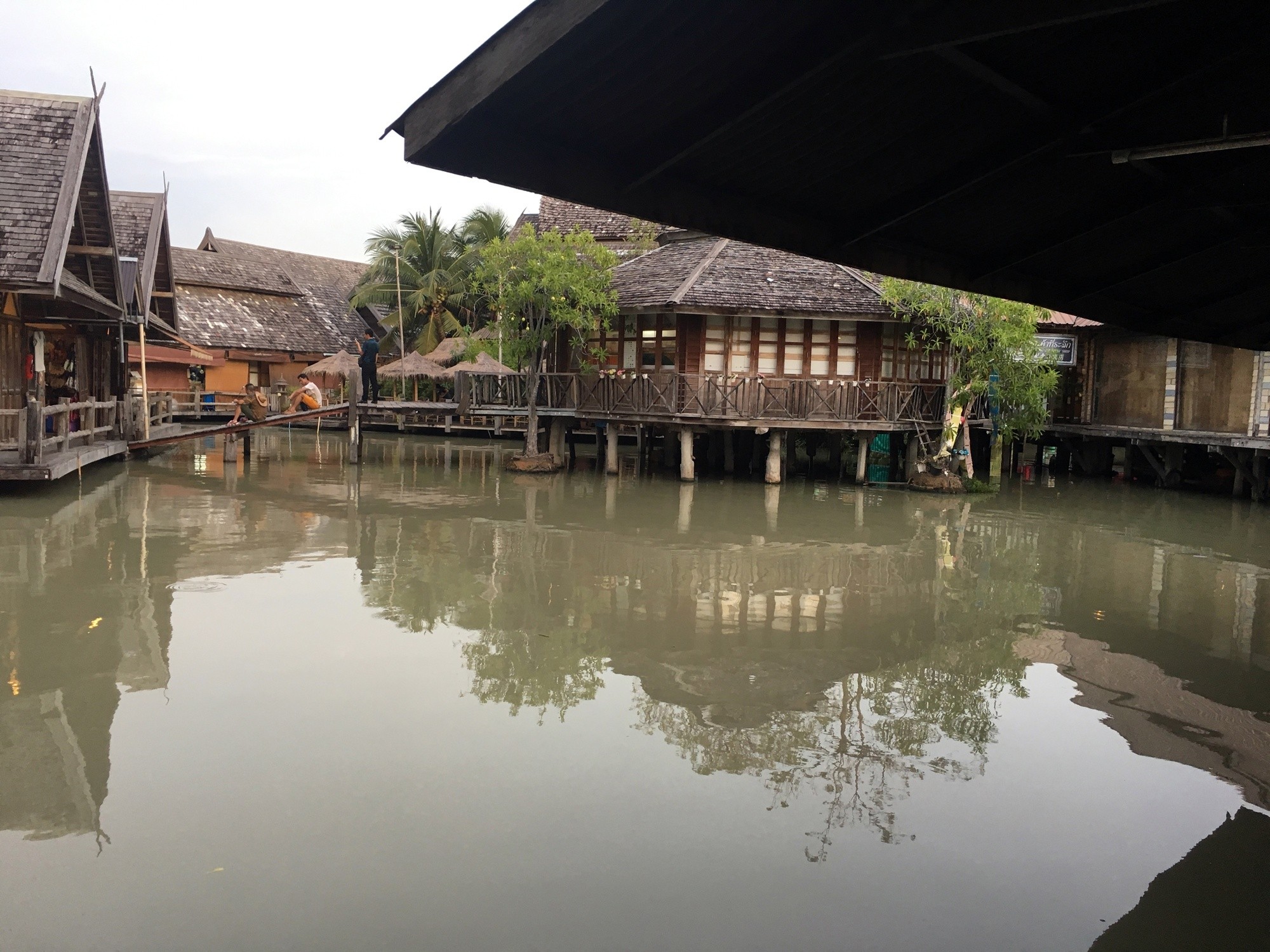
[{"left": 358, "top": 354, "right": 365, "bottom": 367}]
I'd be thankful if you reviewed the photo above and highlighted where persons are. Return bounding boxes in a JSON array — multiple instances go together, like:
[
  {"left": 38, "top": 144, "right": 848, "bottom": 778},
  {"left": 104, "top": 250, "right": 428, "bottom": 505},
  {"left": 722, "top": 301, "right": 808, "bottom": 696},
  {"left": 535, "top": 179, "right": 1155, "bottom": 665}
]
[
  {"left": 283, "top": 373, "right": 322, "bottom": 414},
  {"left": 354, "top": 328, "right": 379, "bottom": 404},
  {"left": 226, "top": 383, "right": 268, "bottom": 426}
]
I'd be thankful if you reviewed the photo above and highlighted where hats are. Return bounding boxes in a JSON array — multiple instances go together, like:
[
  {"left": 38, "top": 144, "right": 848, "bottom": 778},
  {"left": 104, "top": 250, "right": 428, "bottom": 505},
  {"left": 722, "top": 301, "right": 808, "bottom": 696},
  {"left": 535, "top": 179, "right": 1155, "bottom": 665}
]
[{"left": 255, "top": 391, "right": 269, "bottom": 407}]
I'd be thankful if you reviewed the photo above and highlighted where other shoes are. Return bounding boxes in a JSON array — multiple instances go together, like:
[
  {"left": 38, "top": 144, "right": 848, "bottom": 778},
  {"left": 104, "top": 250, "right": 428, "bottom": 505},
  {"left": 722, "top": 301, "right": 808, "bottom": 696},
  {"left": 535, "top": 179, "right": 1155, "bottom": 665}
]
[{"left": 226, "top": 417, "right": 248, "bottom": 426}]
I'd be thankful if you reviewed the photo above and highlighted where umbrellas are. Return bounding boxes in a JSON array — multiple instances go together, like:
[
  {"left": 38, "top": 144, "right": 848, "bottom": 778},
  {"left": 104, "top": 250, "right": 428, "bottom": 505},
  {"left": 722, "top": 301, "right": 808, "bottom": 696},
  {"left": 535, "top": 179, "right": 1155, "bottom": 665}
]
[
  {"left": 377, "top": 350, "right": 452, "bottom": 403},
  {"left": 303, "top": 349, "right": 363, "bottom": 404},
  {"left": 441, "top": 350, "right": 518, "bottom": 402},
  {"left": 420, "top": 334, "right": 477, "bottom": 362}
]
